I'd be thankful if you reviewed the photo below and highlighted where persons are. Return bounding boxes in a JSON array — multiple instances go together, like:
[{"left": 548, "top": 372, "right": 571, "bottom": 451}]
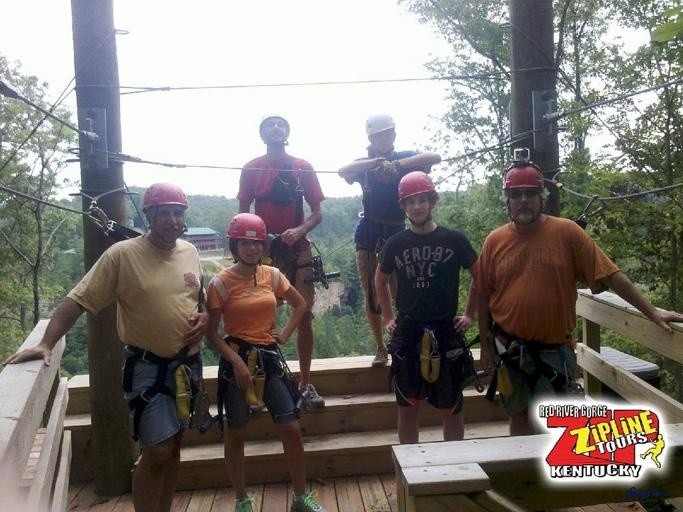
[
  {"left": 374, "top": 171, "right": 481, "bottom": 444},
  {"left": 3, "top": 184, "right": 209, "bottom": 512},
  {"left": 205, "top": 213, "right": 330, "bottom": 512},
  {"left": 339, "top": 113, "right": 440, "bottom": 366},
  {"left": 477, "top": 165, "right": 683, "bottom": 437},
  {"left": 235, "top": 114, "right": 328, "bottom": 409}
]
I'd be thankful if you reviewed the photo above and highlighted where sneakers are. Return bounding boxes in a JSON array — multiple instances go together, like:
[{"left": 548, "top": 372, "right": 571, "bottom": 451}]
[
  {"left": 370, "top": 345, "right": 390, "bottom": 368},
  {"left": 290, "top": 491, "right": 325, "bottom": 511},
  {"left": 295, "top": 383, "right": 325, "bottom": 411},
  {"left": 234, "top": 494, "right": 254, "bottom": 512}
]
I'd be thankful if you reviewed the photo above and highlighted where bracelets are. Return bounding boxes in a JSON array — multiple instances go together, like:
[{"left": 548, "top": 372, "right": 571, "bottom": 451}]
[{"left": 391, "top": 159, "right": 401, "bottom": 170}]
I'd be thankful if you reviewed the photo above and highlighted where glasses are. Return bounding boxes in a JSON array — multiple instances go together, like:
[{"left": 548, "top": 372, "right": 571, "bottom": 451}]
[{"left": 507, "top": 189, "right": 538, "bottom": 198}]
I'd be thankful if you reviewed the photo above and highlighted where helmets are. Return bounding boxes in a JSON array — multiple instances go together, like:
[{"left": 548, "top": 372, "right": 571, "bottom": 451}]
[
  {"left": 141, "top": 182, "right": 189, "bottom": 212},
  {"left": 501, "top": 160, "right": 545, "bottom": 190},
  {"left": 397, "top": 169, "right": 434, "bottom": 204},
  {"left": 226, "top": 212, "right": 267, "bottom": 242},
  {"left": 366, "top": 112, "right": 396, "bottom": 136}
]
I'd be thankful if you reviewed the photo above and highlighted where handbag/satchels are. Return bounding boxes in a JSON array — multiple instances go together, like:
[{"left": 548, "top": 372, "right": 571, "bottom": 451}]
[{"left": 270, "top": 170, "right": 298, "bottom": 206}]
